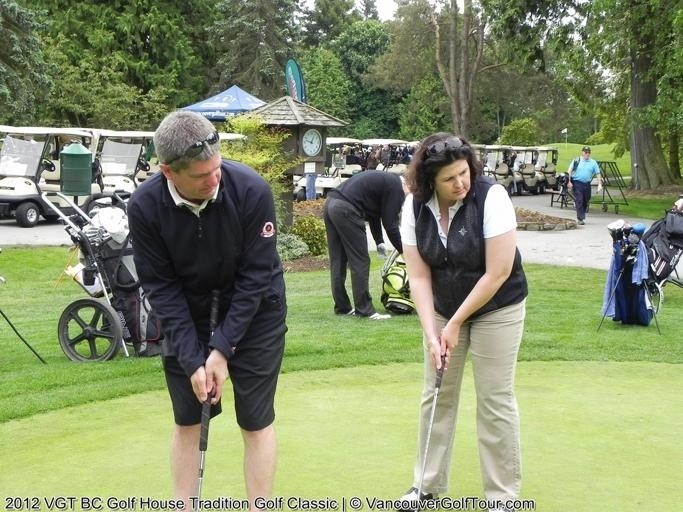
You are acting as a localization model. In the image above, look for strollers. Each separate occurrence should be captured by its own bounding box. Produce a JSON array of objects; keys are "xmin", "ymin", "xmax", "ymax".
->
[{"xmin": 38, "ymin": 191, "xmax": 159, "ymax": 365}]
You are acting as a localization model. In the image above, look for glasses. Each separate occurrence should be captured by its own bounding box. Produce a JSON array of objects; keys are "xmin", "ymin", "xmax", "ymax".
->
[
  {"xmin": 421, "ymin": 136, "xmax": 467, "ymax": 162},
  {"xmin": 584, "ymin": 151, "xmax": 590, "ymax": 154},
  {"xmin": 167, "ymin": 130, "xmax": 219, "ymax": 167}
]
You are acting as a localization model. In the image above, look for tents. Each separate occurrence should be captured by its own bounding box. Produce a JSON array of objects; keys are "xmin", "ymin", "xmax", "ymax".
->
[{"xmin": 180, "ymin": 85, "xmax": 267, "ymax": 120}]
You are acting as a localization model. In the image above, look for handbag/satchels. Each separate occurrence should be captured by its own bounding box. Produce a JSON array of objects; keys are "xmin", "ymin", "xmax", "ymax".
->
[
  {"xmin": 555, "ymin": 172, "xmax": 569, "ymax": 187},
  {"xmin": 381, "ymin": 255, "xmax": 416, "ymax": 314}
]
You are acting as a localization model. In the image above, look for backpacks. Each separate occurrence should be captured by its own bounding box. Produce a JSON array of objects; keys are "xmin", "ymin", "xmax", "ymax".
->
[{"xmin": 376, "ymin": 246, "xmax": 419, "ymax": 315}]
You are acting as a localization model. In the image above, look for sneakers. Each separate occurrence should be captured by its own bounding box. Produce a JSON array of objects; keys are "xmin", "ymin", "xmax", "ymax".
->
[
  {"xmin": 578, "ymin": 220, "xmax": 584, "ymax": 225},
  {"xmin": 399, "ymin": 487, "xmax": 440, "ymax": 512},
  {"xmin": 347, "ymin": 309, "xmax": 356, "ymax": 316},
  {"xmin": 369, "ymin": 313, "xmax": 392, "ymax": 320}
]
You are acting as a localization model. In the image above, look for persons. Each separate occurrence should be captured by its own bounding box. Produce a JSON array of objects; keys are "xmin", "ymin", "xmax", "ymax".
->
[
  {"xmin": 127, "ymin": 112, "xmax": 288, "ymax": 511},
  {"xmin": 567, "ymin": 146, "xmax": 602, "ymax": 225},
  {"xmin": 400, "ymin": 133, "xmax": 529, "ymax": 510},
  {"xmin": 323, "ymin": 170, "xmax": 407, "ymax": 319}
]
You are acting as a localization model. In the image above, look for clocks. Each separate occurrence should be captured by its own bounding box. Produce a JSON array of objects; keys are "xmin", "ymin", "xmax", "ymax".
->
[{"xmin": 302, "ymin": 128, "xmax": 322, "ymax": 156}]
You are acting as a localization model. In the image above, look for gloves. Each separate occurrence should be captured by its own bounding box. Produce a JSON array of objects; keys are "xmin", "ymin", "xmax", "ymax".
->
[{"xmin": 377, "ymin": 243, "xmax": 393, "ymax": 257}]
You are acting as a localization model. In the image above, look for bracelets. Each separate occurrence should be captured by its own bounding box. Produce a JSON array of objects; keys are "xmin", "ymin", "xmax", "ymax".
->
[{"xmin": 427, "ymin": 336, "xmax": 439, "ymax": 348}]
